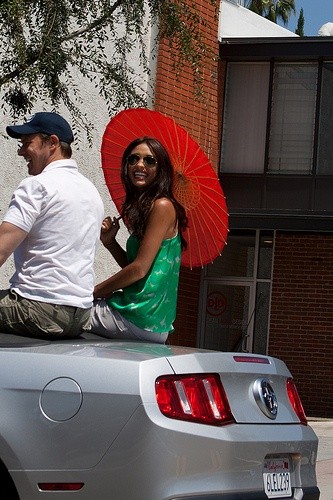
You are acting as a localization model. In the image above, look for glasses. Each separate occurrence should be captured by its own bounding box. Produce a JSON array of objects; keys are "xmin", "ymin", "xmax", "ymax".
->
[{"xmin": 125, "ymin": 154, "xmax": 157, "ymax": 168}]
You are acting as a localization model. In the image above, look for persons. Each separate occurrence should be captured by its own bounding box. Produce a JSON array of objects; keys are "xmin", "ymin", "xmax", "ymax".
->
[
  {"xmin": 88, "ymin": 136, "xmax": 188, "ymax": 346},
  {"xmin": 0, "ymin": 110, "xmax": 104, "ymax": 340}
]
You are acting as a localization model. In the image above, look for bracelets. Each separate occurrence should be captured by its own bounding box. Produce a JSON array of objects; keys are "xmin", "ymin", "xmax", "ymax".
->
[{"xmin": 105, "ymin": 239, "xmax": 116, "ymax": 248}]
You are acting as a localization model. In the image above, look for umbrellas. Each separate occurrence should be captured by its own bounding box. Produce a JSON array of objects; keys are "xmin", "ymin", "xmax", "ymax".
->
[{"xmin": 98, "ymin": 108, "xmax": 231, "ymax": 270}]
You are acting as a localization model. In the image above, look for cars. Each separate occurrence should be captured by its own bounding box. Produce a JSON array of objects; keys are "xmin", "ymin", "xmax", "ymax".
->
[{"xmin": 1, "ymin": 330, "xmax": 321, "ymax": 500}]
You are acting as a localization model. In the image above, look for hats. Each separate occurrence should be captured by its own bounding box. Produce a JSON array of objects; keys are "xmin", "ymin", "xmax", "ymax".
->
[{"xmin": 6, "ymin": 112, "xmax": 74, "ymax": 144}]
